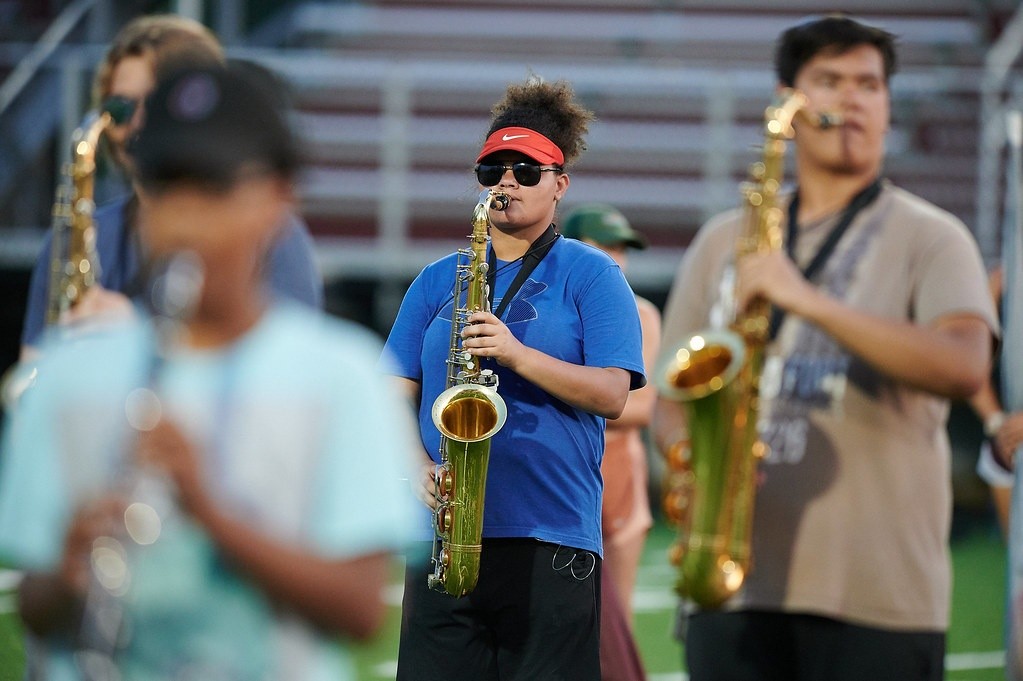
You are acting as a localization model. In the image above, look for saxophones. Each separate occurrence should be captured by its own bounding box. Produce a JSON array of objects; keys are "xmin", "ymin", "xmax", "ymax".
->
[
  {"xmin": 425, "ymin": 185, "xmax": 514, "ymax": 601},
  {"xmin": 44, "ymin": 109, "xmax": 116, "ymax": 331},
  {"xmin": 655, "ymin": 89, "xmax": 815, "ymax": 611}
]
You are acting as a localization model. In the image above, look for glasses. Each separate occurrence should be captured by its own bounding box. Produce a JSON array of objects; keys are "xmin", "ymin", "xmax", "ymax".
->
[
  {"xmin": 474, "ymin": 161, "xmax": 563, "ymax": 187},
  {"xmin": 101, "ymin": 95, "xmax": 152, "ymax": 126}
]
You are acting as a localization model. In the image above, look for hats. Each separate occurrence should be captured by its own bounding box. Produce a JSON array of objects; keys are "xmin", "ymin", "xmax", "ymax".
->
[
  {"xmin": 564, "ymin": 207, "xmax": 647, "ymax": 252},
  {"xmin": 128, "ymin": 58, "xmax": 294, "ymax": 175},
  {"xmin": 476, "ymin": 127, "xmax": 565, "ymax": 167}
]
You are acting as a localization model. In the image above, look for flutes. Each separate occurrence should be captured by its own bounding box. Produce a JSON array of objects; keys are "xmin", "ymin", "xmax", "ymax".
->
[{"xmin": 60, "ymin": 247, "xmax": 203, "ymax": 679}]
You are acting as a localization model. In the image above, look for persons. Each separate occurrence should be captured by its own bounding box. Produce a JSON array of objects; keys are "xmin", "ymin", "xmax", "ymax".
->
[
  {"xmin": 563, "ymin": 203, "xmax": 662, "ymax": 681},
  {"xmin": 379, "ymin": 77, "xmax": 643, "ymax": 681},
  {"xmin": 0, "ymin": 54, "xmax": 431, "ymax": 681},
  {"xmin": 22, "ymin": 11, "xmax": 321, "ymax": 360},
  {"xmin": 971, "ymin": 261, "xmax": 1023, "ymax": 525},
  {"xmin": 653, "ymin": 18, "xmax": 1002, "ymax": 681}
]
